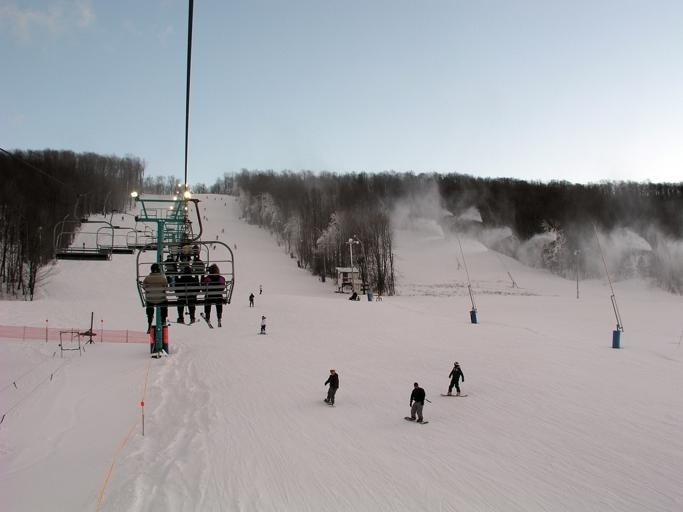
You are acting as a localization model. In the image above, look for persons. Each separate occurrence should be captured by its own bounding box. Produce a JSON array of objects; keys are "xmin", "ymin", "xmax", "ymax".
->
[
  {"xmin": 259, "ymin": 284, "xmax": 263, "ymax": 295},
  {"xmin": 258, "ymin": 315, "xmax": 267, "ymax": 334},
  {"xmin": 322, "ymin": 369, "xmax": 340, "ymax": 406},
  {"xmin": 163, "ymin": 232, "xmax": 209, "ymax": 292},
  {"xmin": 141, "ymin": 262, "xmax": 169, "ymax": 334},
  {"xmin": 248, "ymin": 292, "xmax": 256, "ymax": 307},
  {"xmin": 174, "ymin": 265, "xmax": 200, "ymax": 326},
  {"xmin": 348, "ymin": 289, "xmax": 358, "ymax": 301},
  {"xmin": 201, "ymin": 263, "xmax": 227, "ymax": 323},
  {"xmin": 445, "ymin": 361, "xmax": 465, "ymax": 396},
  {"xmin": 409, "ymin": 382, "xmax": 426, "ymax": 423}
]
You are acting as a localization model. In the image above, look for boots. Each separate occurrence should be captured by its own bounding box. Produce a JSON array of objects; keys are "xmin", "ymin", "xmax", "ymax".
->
[
  {"xmin": 411, "ymin": 417, "xmax": 423, "ymax": 422},
  {"xmin": 326, "ymin": 398, "xmax": 334, "ymax": 404},
  {"xmin": 448, "ymin": 391, "xmax": 460, "ymax": 396}
]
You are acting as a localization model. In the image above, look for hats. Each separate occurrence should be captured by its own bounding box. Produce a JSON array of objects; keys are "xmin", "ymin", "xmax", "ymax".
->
[{"xmin": 455, "ymin": 362, "xmax": 460, "ymax": 365}]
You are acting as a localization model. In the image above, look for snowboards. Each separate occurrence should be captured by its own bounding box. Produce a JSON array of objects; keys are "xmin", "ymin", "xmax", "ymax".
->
[
  {"xmin": 405, "ymin": 417, "xmax": 428, "ymax": 424},
  {"xmin": 440, "ymin": 393, "xmax": 467, "ymax": 397},
  {"xmin": 324, "ymin": 399, "xmax": 333, "ymax": 406}
]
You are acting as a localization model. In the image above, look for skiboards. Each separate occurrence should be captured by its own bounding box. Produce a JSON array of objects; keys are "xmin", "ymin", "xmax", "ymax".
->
[
  {"xmin": 167, "ymin": 316, "xmax": 200, "ymax": 327},
  {"xmin": 147, "ymin": 316, "xmax": 169, "ymax": 334},
  {"xmin": 200, "ymin": 312, "xmax": 222, "ymax": 328}
]
